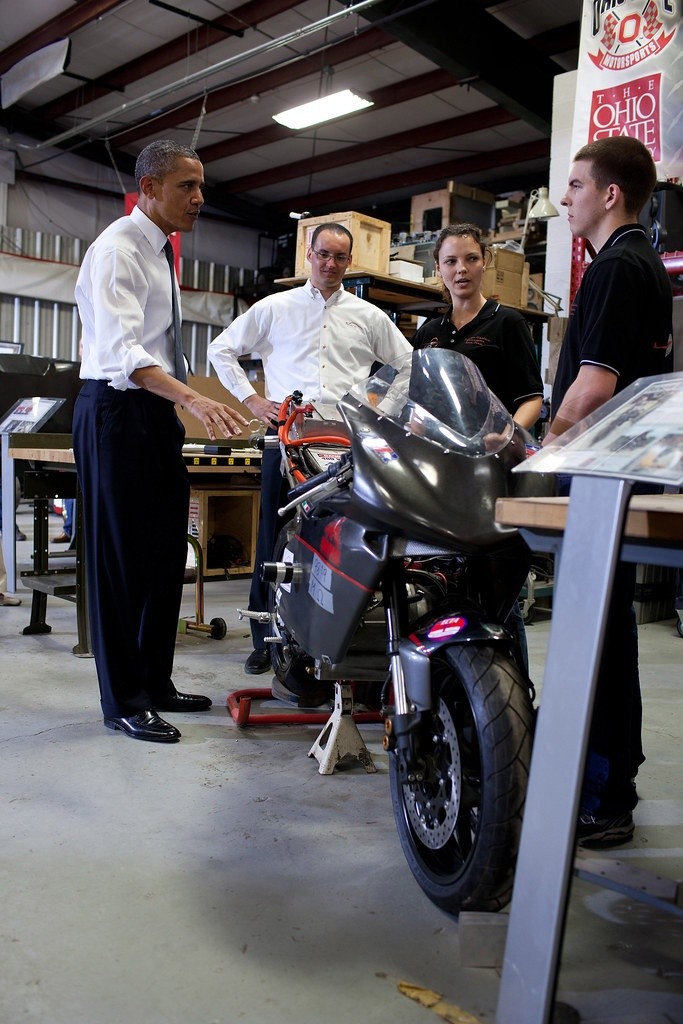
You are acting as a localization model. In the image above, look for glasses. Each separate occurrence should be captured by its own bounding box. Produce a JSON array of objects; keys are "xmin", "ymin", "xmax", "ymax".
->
[{"xmin": 313, "ymin": 251, "xmax": 347, "ymax": 262}]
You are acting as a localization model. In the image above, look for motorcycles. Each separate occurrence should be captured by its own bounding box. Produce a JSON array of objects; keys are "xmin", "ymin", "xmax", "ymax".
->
[{"xmin": 237, "ymin": 348, "xmax": 533, "ymax": 911}]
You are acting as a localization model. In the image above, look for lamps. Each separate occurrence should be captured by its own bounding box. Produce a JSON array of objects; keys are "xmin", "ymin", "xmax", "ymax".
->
[
  {"xmin": 520, "ymin": 187, "xmax": 559, "ymax": 247},
  {"xmin": 270, "ymin": 88, "xmax": 375, "ymax": 130}
]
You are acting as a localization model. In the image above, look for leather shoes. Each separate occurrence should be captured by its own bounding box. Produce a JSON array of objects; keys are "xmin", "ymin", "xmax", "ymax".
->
[
  {"xmin": 103, "ymin": 710, "xmax": 182, "ymax": 742},
  {"xmin": 245, "ymin": 648, "xmax": 271, "ymax": 675},
  {"xmin": 149, "ymin": 690, "xmax": 212, "ymax": 713}
]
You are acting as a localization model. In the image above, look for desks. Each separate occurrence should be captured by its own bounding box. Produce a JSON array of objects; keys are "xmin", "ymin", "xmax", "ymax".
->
[
  {"xmin": 9, "ymin": 448, "xmax": 263, "ymax": 659},
  {"xmin": 496, "ymin": 493, "xmax": 683, "ymax": 595}
]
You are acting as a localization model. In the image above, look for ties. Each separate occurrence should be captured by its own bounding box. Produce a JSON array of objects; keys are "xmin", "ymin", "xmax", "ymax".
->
[{"xmin": 163, "ymin": 240, "xmax": 187, "ymax": 410}]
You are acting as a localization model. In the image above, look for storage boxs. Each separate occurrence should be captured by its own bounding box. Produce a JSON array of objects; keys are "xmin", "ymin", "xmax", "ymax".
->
[
  {"xmin": 480, "ymin": 246, "xmax": 524, "ymax": 307},
  {"xmin": 295, "ymin": 211, "xmax": 392, "ymax": 275},
  {"xmin": 389, "ymin": 260, "xmax": 423, "ymax": 282}
]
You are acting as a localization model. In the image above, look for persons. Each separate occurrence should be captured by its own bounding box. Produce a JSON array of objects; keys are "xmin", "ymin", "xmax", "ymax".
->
[
  {"xmin": 207, "ymin": 223, "xmax": 414, "ymax": 674},
  {"xmin": 578, "ymin": 391, "xmax": 683, "ymax": 473},
  {"xmin": 2, "ymin": 404, "xmax": 36, "ymax": 433},
  {"xmin": 413, "ymin": 224, "xmax": 545, "ymax": 680},
  {"xmin": 540, "ymin": 136, "xmax": 672, "ymax": 847},
  {"xmin": 0, "ymin": 476, "xmax": 27, "ymax": 541},
  {"xmin": 51, "ymin": 499, "xmax": 76, "ymax": 543},
  {"xmin": 74, "ymin": 140, "xmax": 249, "ymax": 742}
]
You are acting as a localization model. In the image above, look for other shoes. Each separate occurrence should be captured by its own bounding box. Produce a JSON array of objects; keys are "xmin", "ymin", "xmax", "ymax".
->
[{"xmin": 575, "ymin": 813, "xmax": 634, "ymax": 850}]
